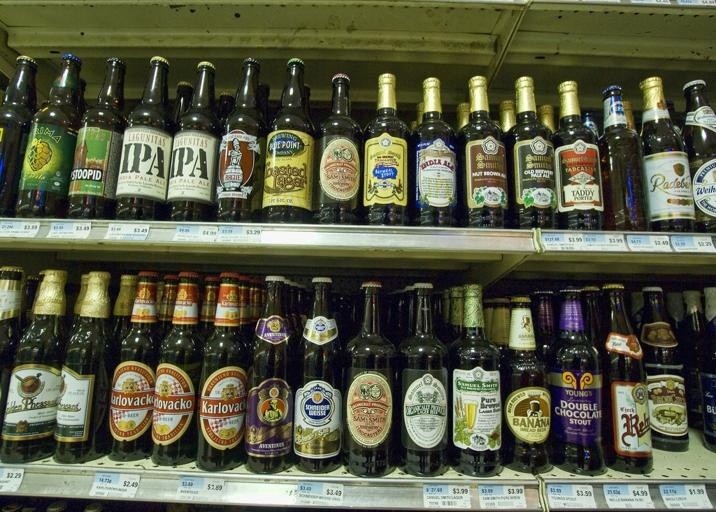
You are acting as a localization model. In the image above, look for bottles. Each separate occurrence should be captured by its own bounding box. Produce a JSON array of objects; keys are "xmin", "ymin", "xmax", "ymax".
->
[
  {"xmin": 622, "ymin": 100, "xmax": 634, "ymax": 131},
  {"xmin": 412, "ymin": 77, "xmax": 458, "ymax": 226},
  {"xmin": 197, "ymin": 273, "xmax": 250, "ymax": 472},
  {"xmin": 502, "ymin": 296, "xmax": 553, "ymax": 475},
  {"xmin": 110, "ymin": 272, "xmax": 161, "ymax": 462},
  {"xmin": 66, "ymin": 57, "xmax": 125, "ymax": 219},
  {"xmin": 152, "ymin": 272, "xmax": 203, "ymax": 466},
  {"xmin": 55, "ymin": 272, "xmax": 113, "ymax": 464},
  {"xmin": 238, "ymin": 275, "xmax": 250, "ymax": 351},
  {"xmin": 71, "ymin": 275, "xmax": 88, "ymax": 337},
  {"xmin": 21, "ymin": 276, "xmax": 40, "ymax": 338},
  {"xmin": 158, "ymin": 276, "xmax": 179, "ymax": 348},
  {"xmin": 505, "ymin": 77, "xmax": 554, "ymax": 228},
  {"xmin": 417, "ymin": 102, "xmax": 425, "ymax": 127},
  {"xmin": 581, "ymin": 287, "xmax": 603, "ymax": 349},
  {"xmin": 640, "ymin": 77, "xmax": 695, "ymax": 232},
  {"xmin": 343, "ymin": 282, "xmax": 398, "ymax": 478},
  {"xmin": 262, "ymin": 59, "xmax": 316, "ymax": 223},
  {"xmin": 0, "ymin": 270, "xmax": 66, "ymax": 464},
  {"xmin": 481, "ymin": 299, "xmax": 494, "ymax": 344},
  {"xmin": 315, "ymin": 74, "xmax": 364, "ymax": 223},
  {"xmin": 217, "ymin": 91, "xmax": 236, "ymax": 132},
  {"xmin": 449, "ymin": 287, "xmax": 464, "ymax": 344},
  {"xmin": 582, "ymin": 112, "xmax": 599, "ymax": 139},
  {"xmin": 292, "ymin": 278, "xmax": 344, "ymax": 474},
  {"xmin": 458, "ymin": 103, "xmax": 470, "ymax": 131},
  {"xmin": 363, "ymin": 74, "xmax": 412, "ymax": 225},
  {"xmin": 0, "ymin": 56, "xmax": 38, "ymax": 217},
  {"xmin": 684, "ymin": 292, "xmax": 707, "ymax": 429},
  {"xmin": 215, "ymin": 58, "xmax": 265, "ymax": 222},
  {"xmin": 553, "ymin": 287, "xmax": 605, "ymax": 475},
  {"xmin": 641, "ymin": 288, "xmax": 690, "ymax": 452},
  {"xmin": 665, "ymin": 288, "xmax": 685, "ymax": 334},
  {"xmin": 538, "ymin": 105, "xmax": 555, "ymax": 133},
  {"xmin": 500, "ymin": 100, "xmax": 517, "ymax": 133},
  {"xmin": 555, "ymin": 81, "xmax": 604, "ymax": 229},
  {"xmin": 244, "ymin": 276, "xmax": 293, "ymax": 475},
  {"xmin": 603, "ymin": 284, "xmax": 653, "ymax": 474},
  {"xmin": 681, "ymin": 80, "xmax": 716, "ymax": 232},
  {"xmin": 532, "ymin": 290, "xmax": 555, "ymax": 354},
  {"xmin": 113, "ymin": 275, "xmax": 137, "ymax": 366},
  {"xmin": 198, "ymin": 276, "xmax": 219, "ymax": 341},
  {"xmin": 397, "ymin": 283, "xmax": 451, "ymax": 476},
  {"xmin": 166, "ymin": 62, "xmax": 221, "ymax": 221},
  {"xmin": 172, "ymin": 82, "xmax": 195, "ymax": 126},
  {"xmin": 449, "ymin": 285, "xmax": 505, "ymax": 477},
  {"xmin": 0, "ymin": 265, "xmax": 22, "ymax": 435},
  {"xmin": 490, "ymin": 299, "xmax": 511, "ymax": 352},
  {"xmin": 115, "ymin": 56, "xmax": 174, "ymax": 220},
  {"xmin": 699, "ymin": 287, "xmax": 716, "ymax": 452},
  {"xmin": 16, "ymin": 54, "xmax": 80, "ymax": 218},
  {"xmin": 458, "ymin": 76, "xmax": 507, "ymax": 228},
  {"xmin": 596, "ymin": 85, "xmax": 649, "ymax": 231}
]
